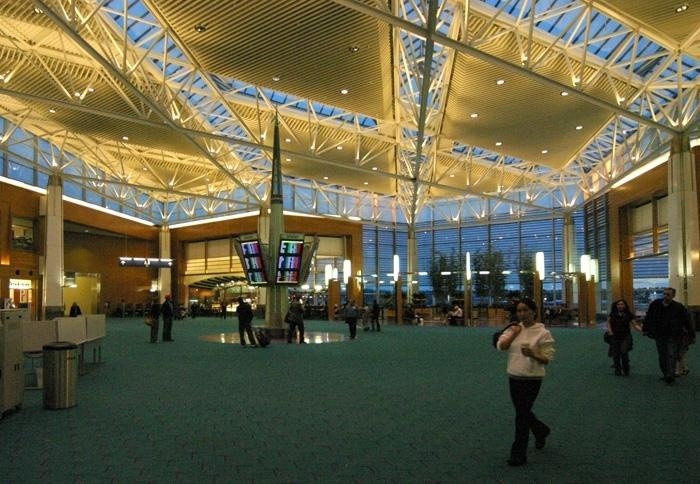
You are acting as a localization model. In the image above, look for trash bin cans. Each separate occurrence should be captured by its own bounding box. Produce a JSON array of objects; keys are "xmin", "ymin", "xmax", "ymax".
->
[{"xmin": 42, "ymin": 341, "xmax": 78, "ymax": 409}]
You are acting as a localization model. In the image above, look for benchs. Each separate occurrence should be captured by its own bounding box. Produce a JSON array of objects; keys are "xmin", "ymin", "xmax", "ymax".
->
[{"xmin": 384, "ymin": 302, "xmax": 511, "ymax": 325}]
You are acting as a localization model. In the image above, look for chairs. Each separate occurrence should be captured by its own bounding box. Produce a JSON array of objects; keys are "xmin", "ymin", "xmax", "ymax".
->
[{"xmin": 21, "ymin": 312, "xmax": 107, "ymax": 390}]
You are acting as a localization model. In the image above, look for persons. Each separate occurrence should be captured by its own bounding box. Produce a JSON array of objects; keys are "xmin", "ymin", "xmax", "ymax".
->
[
  {"xmin": 286, "ymin": 292, "xmax": 307, "ymax": 345},
  {"xmin": 343, "ymin": 298, "xmax": 380, "ymax": 340},
  {"xmin": 69, "ymin": 302, "xmax": 81, "ymax": 317},
  {"xmin": 160, "ymin": 293, "xmax": 175, "ymax": 341},
  {"xmin": 149, "ymin": 299, "xmax": 161, "ymax": 343},
  {"xmin": 497, "ymin": 298, "xmax": 556, "ymax": 467},
  {"xmin": 220, "ymin": 299, "xmax": 227, "ymax": 319},
  {"xmin": 448, "ymin": 305, "xmax": 463, "ymax": 326},
  {"xmin": 190, "ymin": 302, "xmax": 197, "ymax": 318},
  {"xmin": 235, "ymin": 296, "xmax": 256, "ymax": 348},
  {"xmin": 606, "ymin": 287, "xmax": 696, "ymax": 385}
]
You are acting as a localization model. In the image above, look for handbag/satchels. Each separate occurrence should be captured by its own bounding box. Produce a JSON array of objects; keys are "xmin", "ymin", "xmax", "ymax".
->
[
  {"xmin": 493, "ymin": 324, "xmax": 521, "ymax": 349},
  {"xmin": 604, "ymin": 331, "xmax": 614, "ymax": 344},
  {"xmin": 284, "ymin": 311, "xmax": 290, "ymax": 324}
]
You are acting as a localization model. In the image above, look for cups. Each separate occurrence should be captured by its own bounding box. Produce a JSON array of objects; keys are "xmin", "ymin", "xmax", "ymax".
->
[{"xmin": 520, "ymin": 341, "xmax": 529, "ymax": 348}]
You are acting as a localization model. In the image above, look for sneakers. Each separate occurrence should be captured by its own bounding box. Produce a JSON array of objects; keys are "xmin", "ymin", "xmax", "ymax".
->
[
  {"xmin": 507, "ymin": 455, "xmax": 528, "ymax": 467},
  {"xmin": 535, "ymin": 426, "xmax": 551, "ymax": 450}
]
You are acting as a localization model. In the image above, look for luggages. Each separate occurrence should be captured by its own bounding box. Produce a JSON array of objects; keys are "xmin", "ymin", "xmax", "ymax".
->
[{"xmin": 255, "ymin": 328, "xmax": 271, "ymax": 348}]
